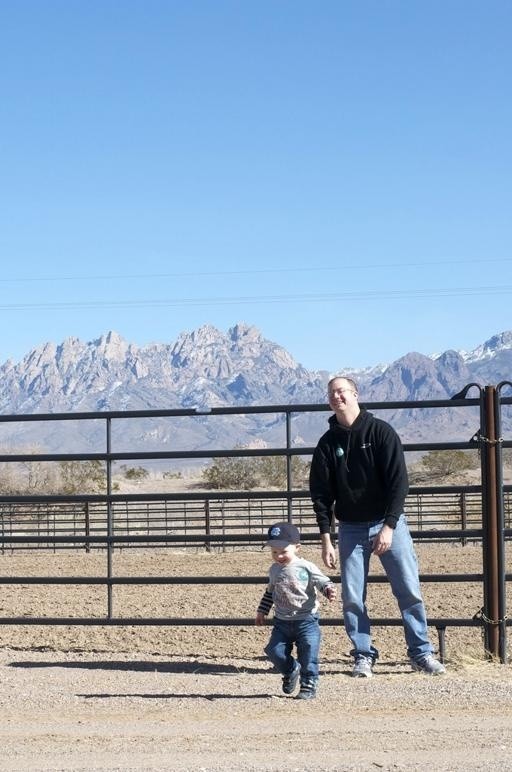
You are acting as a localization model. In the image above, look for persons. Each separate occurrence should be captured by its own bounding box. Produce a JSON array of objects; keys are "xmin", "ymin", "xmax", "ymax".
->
[
  {"xmin": 310, "ymin": 375, "xmax": 446, "ymax": 676},
  {"xmin": 255, "ymin": 521, "xmax": 337, "ymax": 701}
]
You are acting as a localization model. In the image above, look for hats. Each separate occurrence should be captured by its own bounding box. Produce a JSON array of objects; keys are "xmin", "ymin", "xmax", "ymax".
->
[{"xmin": 261, "ymin": 522, "xmax": 300, "ymax": 550}]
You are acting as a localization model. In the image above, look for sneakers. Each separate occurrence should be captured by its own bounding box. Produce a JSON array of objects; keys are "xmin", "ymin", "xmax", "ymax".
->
[
  {"xmin": 352, "ymin": 655, "xmax": 373, "ymax": 678},
  {"xmin": 295, "ymin": 686, "xmax": 316, "ymax": 698},
  {"xmin": 412, "ymin": 654, "xmax": 446, "ymax": 674},
  {"xmin": 282, "ymin": 661, "xmax": 301, "ymax": 692}
]
